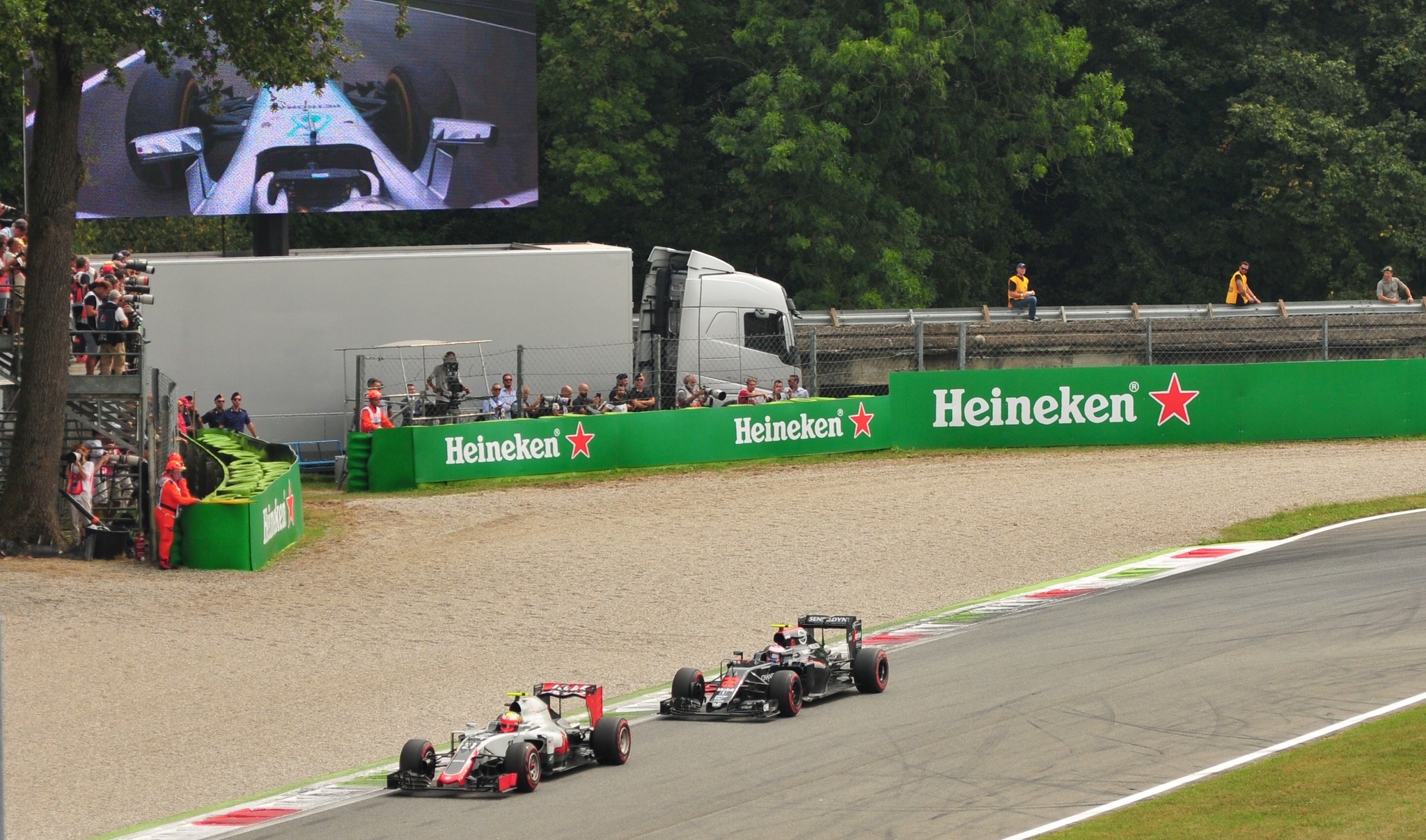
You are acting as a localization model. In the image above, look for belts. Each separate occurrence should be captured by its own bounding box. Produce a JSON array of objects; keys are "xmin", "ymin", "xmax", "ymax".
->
[{"xmin": 159, "ymin": 503, "xmax": 176, "ymax": 512}]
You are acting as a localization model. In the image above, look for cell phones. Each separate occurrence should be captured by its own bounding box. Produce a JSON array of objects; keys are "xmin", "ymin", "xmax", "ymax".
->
[{"xmin": 596, "ymin": 392, "xmax": 601, "ymax": 399}]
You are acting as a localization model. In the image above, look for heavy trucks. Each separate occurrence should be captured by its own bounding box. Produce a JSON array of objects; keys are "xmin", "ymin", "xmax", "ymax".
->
[{"xmin": 136, "ymin": 239, "xmax": 809, "ymax": 463}]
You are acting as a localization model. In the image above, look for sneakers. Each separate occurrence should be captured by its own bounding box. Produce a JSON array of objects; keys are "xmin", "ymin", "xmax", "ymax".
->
[{"xmin": 1027, "ymin": 316, "xmax": 1041, "ymax": 322}]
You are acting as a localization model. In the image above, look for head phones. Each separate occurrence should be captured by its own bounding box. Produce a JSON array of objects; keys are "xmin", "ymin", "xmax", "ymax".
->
[{"xmin": 442, "ymin": 352, "xmax": 459, "ymax": 361}]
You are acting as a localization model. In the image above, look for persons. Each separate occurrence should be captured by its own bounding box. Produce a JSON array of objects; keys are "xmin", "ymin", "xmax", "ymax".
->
[
  {"xmin": 0, "ymin": 202, "xmax": 28, "ymax": 335},
  {"xmin": 783, "ymin": 375, "xmax": 809, "ymax": 400},
  {"xmin": 426, "ymin": 351, "xmax": 471, "ymax": 425},
  {"xmin": 69, "ymin": 250, "xmax": 139, "ymax": 375},
  {"xmin": 482, "ymin": 374, "xmax": 541, "ymax": 421},
  {"xmin": 1226, "ymin": 261, "xmax": 1261, "ymax": 307},
  {"xmin": 176, "ymin": 392, "xmax": 259, "ymax": 444},
  {"xmin": 736, "ymin": 378, "xmax": 769, "ymax": 404},
  {"xmin": 552, "ymin": 373, "xmax": 655, "ymax": 416},
  {"xmin": 359, "ymin": 378, "xmax": 396, "ymax": 433},
  {"xmin": 676, "ymin": 374, "xmax": 709, "ymax": 409},
  {"xmin": 154, "ymin": 452, "xmax": 200, "ymax": 572},
  {"xmin": 1377, "ymin": 266, "xmax": 1414, "ymax": 304},
  {"xmin": 1008, "ymin": 263, "xmax": 1042, "ymax": 323},
  {"xmin": 400, "ymin": 383, "xmax": 423, "ymax": 428},
  {"xmin": 65, "ymin": 392, "xmax": 153, "ymax": 546},
  {"xmin": 766, "ymin": 380, "xmax": 787, "ymax": 402},
  {"xmin": 500, "ymin": 711, "xmax": 520, "ymax": 733},
  {"xmin": 766, "ymin": 645, "xmax": 785, "ymax": 664}
]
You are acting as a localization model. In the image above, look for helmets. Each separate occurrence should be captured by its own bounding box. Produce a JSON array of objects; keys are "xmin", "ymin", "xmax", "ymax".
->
[
  {"xmin": 368, "ymin": 390, "xmax": 381, "ymax": 399},
  {"xmin": 165, "ymin": 453, "xmax": 186, "ymax": 471},
  {"xmin": 500, "ymin": 712, "xmax": 520, "ymax": 731},
  {"xmin": 766, "ymin": 645, "xmax": 786, "ymax": 663}
]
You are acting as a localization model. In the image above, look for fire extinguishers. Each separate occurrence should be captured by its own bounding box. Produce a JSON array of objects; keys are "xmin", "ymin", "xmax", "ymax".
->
[{"xmin": 134, "ymin": 535, "xmax": 146, "ymax": 560}]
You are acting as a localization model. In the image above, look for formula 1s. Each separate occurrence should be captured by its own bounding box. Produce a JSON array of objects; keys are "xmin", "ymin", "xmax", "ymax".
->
[
  {"xmin": 385, "ymin": 680, "xmax": 631, "ymax": 795},
  {"xmin": 658, "ymin": 614, "xmax": 890, "ymax": 719}
]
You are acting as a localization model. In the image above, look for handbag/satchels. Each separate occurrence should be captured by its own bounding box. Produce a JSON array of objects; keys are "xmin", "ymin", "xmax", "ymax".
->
[{"xmin": 67, "ymin": 472, "xmax": 83, "ymax": 494}]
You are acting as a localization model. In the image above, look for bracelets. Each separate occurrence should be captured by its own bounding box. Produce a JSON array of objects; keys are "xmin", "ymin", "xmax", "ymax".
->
[
  {"xmin": 196, "ymin": 414, "xmax": 199, "ymax": 417},
  {"xmin": 1021, "ymin": 293, "xmax": 1023, "ymax": 297},
  {"xmin": 1253, "ymin": 296, "xmax": 1256, "ymax": 299},
  {"xmin": 256, "ymin": 436, "xmax": 259, "ymax": 438},
  {"xmin": 1408, "ymin": 295, "xmax": 1412, "ymax": 297}
]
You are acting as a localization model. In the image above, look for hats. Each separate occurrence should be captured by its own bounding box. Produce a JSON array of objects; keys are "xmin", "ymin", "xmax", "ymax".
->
[
  {"xmin": 1017, "ymin": 263, "xmax": 1027, "ymax": 268},
  {"xmin": 214, "ymin": 394, "xmax": 222, "ymax": 401},
  {"xmin": 179, "ymin": 397, "xmax": 191, "ymax": 407},
  {"xmin": 616, "ymin": 374, "xmax": 628, "ymax": 379},
  {"xmin": 186, "ymin": 396, "xmax": 192, "ymax": 401},
  {"xmin": 1381, "ymin": 266, "xmax": 1393, "ymax": 273},
  {"xmin": 231, "ymin": 392, "xmax": 239, "ymax": 400},
  {"xmin": 108, "ymin": 289, "xmax": 122, "ymax": 298},
  {"xmin": 103, "ymin": 250, "xmax": 130, "ymax": 284},
  {"xmin": 634, "ymin": 373, "xmax": 643, "ymax": 379}
]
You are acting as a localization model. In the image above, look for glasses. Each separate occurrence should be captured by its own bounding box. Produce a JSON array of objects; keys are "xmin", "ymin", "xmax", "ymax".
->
[
  {"xmin": 493, "ymin": 388, "xmax": 501, "ymax": 391},
  {"xmin": 233, "ymin": 398, "xmax": 241, "ymax": 401},
  {"xmin": 505, "ymin": 379, "xmax": 512, "ymax": 381},
  {"xmin": 1241, "ymin": 267, "xmax": 1248, "ymax": 271},
  {"xmin": 216, "ymin": 400, "xmax": 224, "ymax": 402},
  {"xmin": 637, "ymin": 379, "xmax": 643, "ymax": 381}
]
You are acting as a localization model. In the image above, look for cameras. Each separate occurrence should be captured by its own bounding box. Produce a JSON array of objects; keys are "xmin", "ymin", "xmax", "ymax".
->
[
  {"xmin": 66, "ymin": 451, "xmax": 83, "ymax": 464},
  {"xmin": 4, "ymin": 205, "xmax": 18, "ymax": 215},
  {"xmin": 124, "ymin": 284, "xmax": 151, "ymax": 294},
  {"xmin": 106, "ymin": 453, "xmax": 140, "ymax": 465},
  {"xmin": 123, "ymin": 293, "xmax": 154, "ymax": 305},
  {"xmin": 700, "ymin": 385, "xmax": 727, "ymax": 401},
  {"xmin": 122, "ymin": 258, "xmax": 156, "ymax": 274},
  {"xmin": 541, "ymin": 395, "xmax": 571, "ymax": 406},
  {"xmin": 124, "ymin": 275, "xmax": 149, "ymax": 286}
]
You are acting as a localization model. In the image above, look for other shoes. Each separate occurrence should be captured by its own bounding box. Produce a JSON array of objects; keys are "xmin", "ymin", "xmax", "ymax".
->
[
  {"xmin": 161, "ymin": 562, "xmax": 176, "ymax": 570},
  {"xmin": 76, "ymin": 354, "xmax": 89, "ymax": 363},
  {"xmin": 71, "ymin": 541, "xmax": 78, "ymax": 546},
  {"xmin": 80, "ymin": 538, "xmax": 86, "ymax": 545}
]
